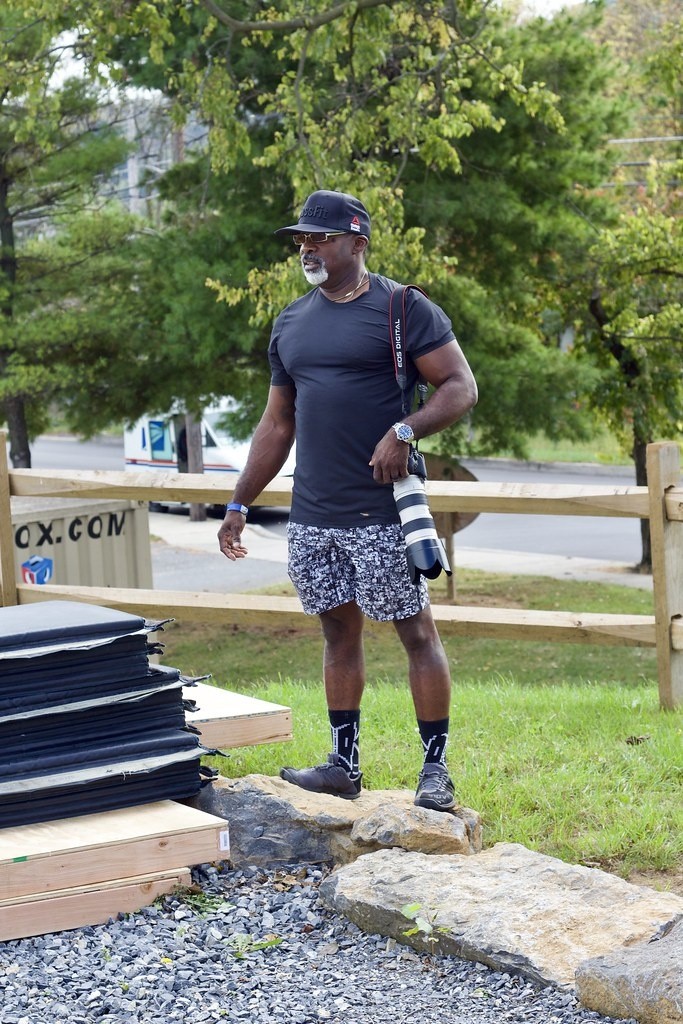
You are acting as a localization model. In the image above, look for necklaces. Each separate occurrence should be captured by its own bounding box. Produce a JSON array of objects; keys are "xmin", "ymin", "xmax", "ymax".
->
[{"xmin": 332, "ymin": 270, "xmax": 370, "ymax": 303}]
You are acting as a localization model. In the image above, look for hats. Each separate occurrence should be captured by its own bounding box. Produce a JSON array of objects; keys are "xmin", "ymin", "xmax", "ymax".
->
[{"xmin": 274, "ymin": 189, "xmax": 371, "ymax": 240}]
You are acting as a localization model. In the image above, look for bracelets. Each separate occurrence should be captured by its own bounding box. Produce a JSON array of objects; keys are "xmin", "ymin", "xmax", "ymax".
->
[{"xmin": 226, "ymin": 503, "xmax": 248, "ymax": 516}]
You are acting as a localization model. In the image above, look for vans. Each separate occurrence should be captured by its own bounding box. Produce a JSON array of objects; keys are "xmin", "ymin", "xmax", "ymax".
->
[{"xmin": 124, "ymin": 391, "xmax": 297, "ymax": 517}]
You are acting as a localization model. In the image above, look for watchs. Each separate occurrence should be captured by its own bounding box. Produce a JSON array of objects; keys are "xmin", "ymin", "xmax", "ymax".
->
[{"xmin": 390, "ymin": 422, "xmax": 415, "ymax": 445}]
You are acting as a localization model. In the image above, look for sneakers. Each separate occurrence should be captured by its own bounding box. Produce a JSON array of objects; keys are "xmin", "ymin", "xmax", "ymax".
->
[
  {"xmin": 414, "ymin": 763, "xmax": 455, "ymax": 811},
  {"xmin": 280, "ymin": 753, "xmax": 363, "ymax": 799}
]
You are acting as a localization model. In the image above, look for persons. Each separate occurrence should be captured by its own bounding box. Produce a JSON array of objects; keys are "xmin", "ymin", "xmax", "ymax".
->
[{"xmin": 218, "ymin": 191, "xmax": 480, "ymax": 811}]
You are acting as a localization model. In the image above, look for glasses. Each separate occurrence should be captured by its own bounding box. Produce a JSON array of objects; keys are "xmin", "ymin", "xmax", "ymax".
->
[{"xmin": 293, "ymin": 232, "xmax": 349, "ymax": 245}]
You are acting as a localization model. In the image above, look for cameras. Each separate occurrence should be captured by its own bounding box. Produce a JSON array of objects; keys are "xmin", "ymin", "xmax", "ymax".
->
[{"xmin": 391, "ymin": 449, "xmax": 453, "ymax": 585}]
